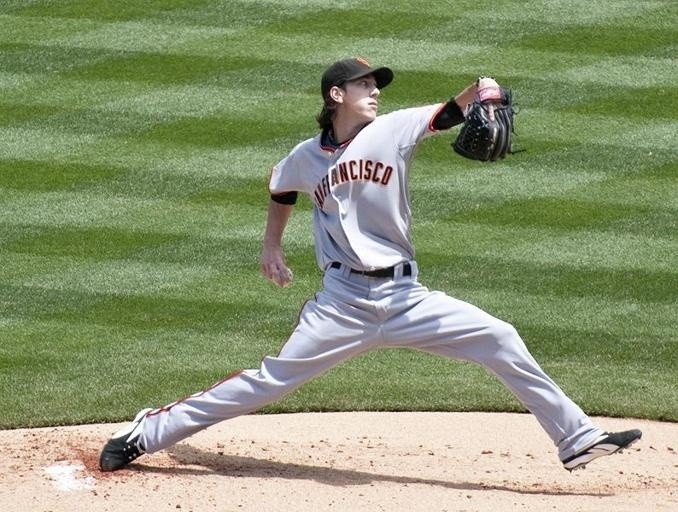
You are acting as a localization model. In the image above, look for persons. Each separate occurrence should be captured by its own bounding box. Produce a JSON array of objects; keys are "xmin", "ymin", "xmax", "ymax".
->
[{"xmin": 95, "ymin": 56, "xmax": 643, "ymax": 478}]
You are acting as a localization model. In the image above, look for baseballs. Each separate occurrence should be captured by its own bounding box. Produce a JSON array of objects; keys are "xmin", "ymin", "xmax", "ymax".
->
[{"xmin": 273, "ymin": 268, "xmax": 292, "ymax": 286}]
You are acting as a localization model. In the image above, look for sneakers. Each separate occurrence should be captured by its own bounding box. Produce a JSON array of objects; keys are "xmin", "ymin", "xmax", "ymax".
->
[
  {"xmin": 562, "ymin": 429, "xmax": 642, "ymax": 472},
  {"xmin": 99, "ymin": 407, "xmax": 155, "ymax": 472}
]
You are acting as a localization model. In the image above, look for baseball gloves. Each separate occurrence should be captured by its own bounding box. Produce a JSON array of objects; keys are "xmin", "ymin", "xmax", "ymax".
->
[{"xmin": 451, "ymin": 76, "xmax": 527, "ymax": 162}]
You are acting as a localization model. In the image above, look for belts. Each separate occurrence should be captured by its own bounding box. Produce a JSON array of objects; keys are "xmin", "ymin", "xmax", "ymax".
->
[{"xmin": 330, "ymin": 261, "xmax": 421, "ymax": 277}]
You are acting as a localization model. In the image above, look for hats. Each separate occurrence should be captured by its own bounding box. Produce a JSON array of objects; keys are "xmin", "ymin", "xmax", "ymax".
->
[{"xmin": 321, "ymin": 56, "xmax": 394, "ymax": 101}]
